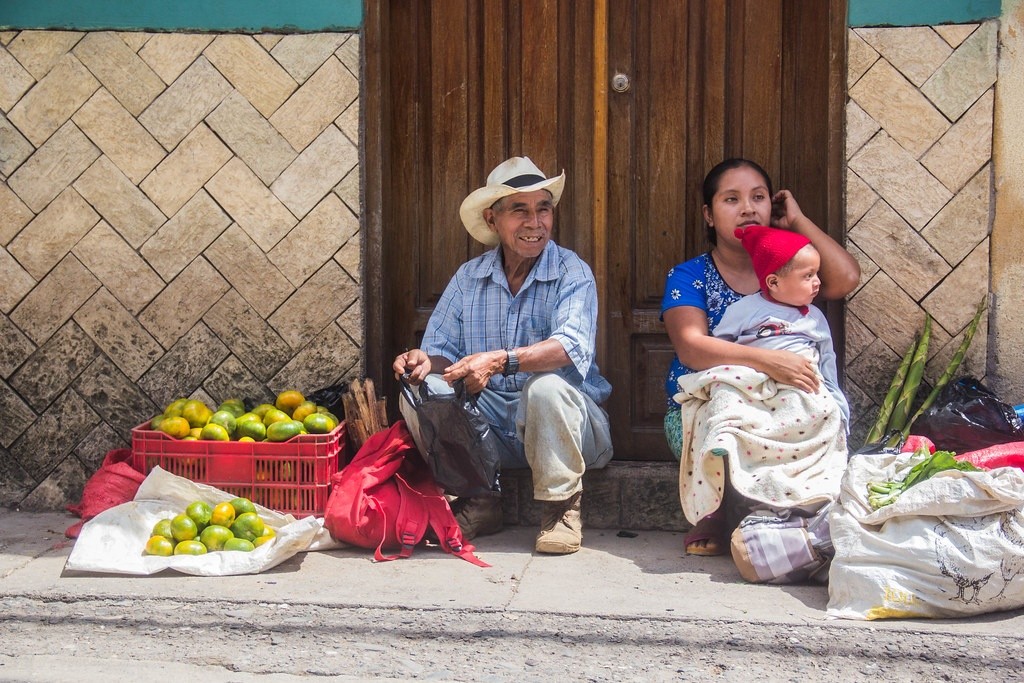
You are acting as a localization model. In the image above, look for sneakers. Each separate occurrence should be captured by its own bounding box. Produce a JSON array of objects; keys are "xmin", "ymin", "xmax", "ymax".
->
[
  {"xmin": 535, "ymin": 489, "xmax": 582, "ymax": 553},
  {"xmin": 429, "ymin": 498, "xmax": 504, "ymax": 541}
]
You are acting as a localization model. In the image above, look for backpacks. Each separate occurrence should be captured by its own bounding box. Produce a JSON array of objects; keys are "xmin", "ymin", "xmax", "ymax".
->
[{"xmin": 324, "ymin": 420, "xmax": 476, "ymax": 567}]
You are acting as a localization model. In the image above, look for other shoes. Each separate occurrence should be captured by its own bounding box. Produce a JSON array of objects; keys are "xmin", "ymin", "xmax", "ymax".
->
[{"xmin": 684, "ymin": 511, "xmax": 729, "ymax": 555}]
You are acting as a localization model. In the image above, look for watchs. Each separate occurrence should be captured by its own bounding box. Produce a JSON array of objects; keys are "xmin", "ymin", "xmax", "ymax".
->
[{"xmin": 502, "ymin": 346, "xmax": 520, "ymax": 378}]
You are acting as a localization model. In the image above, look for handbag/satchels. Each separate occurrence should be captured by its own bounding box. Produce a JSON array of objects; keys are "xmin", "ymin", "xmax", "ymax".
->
[{"xmin": 731, "ymin": 510, "xmax": 831, "ymax": 583}]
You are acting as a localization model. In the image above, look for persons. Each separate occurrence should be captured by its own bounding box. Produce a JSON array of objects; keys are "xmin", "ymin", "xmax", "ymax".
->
[
  {"xmin": 658, "ymin": 157, "xmax": 860, "ymax": 556},
  {"xmin": 711, "ymin": 228, "xmax": 851, "ymax": 456},
  {"xmin": 392, "ymin": 157, "xmax": 616, "ymax": 556}
]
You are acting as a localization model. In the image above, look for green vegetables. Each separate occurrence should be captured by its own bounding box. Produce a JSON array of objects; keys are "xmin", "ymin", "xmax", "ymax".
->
[{"xmin": 867, "ymin": 451, "xmax": 984, "ymax": 511}]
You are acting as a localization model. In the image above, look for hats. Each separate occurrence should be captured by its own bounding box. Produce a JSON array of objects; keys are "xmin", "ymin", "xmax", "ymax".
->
[
  {"xmin": 734, "ymin": 226, "xmax": 812, "ymax": 316},
  {"xmin": 459, "ymin": 156, "xmax": 566, "ymax": 246}
]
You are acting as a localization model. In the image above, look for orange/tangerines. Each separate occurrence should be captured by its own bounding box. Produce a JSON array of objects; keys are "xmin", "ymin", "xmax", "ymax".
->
[
  {"xmin": 146, "ymin": 496, "xmax": 275, "ymax": 557},
  {"xmin": 151, "ymin": 390, "xmax": 339, "ymax": 509}
]
